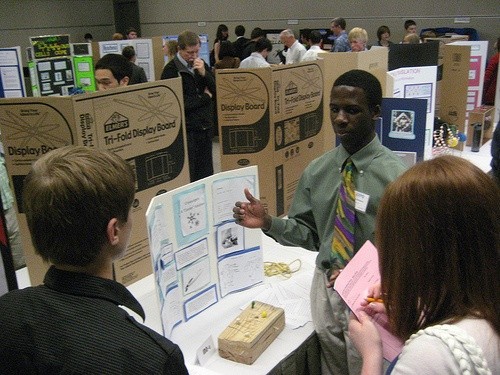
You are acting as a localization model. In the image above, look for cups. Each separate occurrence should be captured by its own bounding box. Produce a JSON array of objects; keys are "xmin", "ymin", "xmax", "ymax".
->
[{"xmin": 471, "ymin": 122, "xmax": 482, "ymax": 152}]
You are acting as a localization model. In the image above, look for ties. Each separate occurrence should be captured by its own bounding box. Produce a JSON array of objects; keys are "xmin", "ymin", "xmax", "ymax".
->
[
  {"xmin": 330, "ymin": 161, "xmax": 356, "ymax": 270},
  {"xmin": 188, "ymin": 63, "xmax": 199, "ymax": 79}
]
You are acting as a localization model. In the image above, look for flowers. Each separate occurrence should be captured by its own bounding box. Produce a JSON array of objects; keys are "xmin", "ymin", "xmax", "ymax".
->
[{"xmin": 432, "ymin": 123, "xmax": 466, "ymax": 158}]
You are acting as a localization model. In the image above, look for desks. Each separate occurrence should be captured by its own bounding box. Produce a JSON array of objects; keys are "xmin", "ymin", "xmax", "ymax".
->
[{"xmin": 16, "ymin": 139, "xmax": 492, "ymax": 375}]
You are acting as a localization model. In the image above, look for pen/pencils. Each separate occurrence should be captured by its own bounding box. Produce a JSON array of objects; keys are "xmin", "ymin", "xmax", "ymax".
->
[{"xmin": 363, "ymin": 297, "xmax": 384, "ymax": 303}]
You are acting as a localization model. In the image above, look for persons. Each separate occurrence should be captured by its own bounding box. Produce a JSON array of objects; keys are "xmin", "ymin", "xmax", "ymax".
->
[
  {"xmin": 279, "ymin": 17, "xmax": 436, "ymax": 65},
  {"xmin": 232, "ymin": 69, "xmax": 411, "ymax": 375},
  {"xmin": 0, "ymin": 146, "xmax": 189, "ymax": 375},
  {"xmin": 84, "ymin": 32, "xmax": 101, "ymax": 68},
  {"xmin": 122, "ymin": 46, "xmax": 148, "ymax": 86},
  {"xmin": 160, "ymin": 32, "xmax": 217, "ymax": 183},
  {"xmin": 347, "ymin": 155, "xmax": 500, "ymax": 375},
  {"xmin": 486, "ymin": 119, "xmax": 500, "ymax": 181},
  {"xmin": 94, "ymin": 53, "xmax": 132, "ymax": 91},
  {"xmin": 112, "ymin": 27, "xmax": 138, "ymax": 43},
  {"xmin": 207, "ymin": 24, "xmax": 273, "ymax": 79},
  {"xmin": 481, "ymin": 37, "xmax": 500, "ymax": 106},
  {"xmin": 163, "ymin": 38, "xmax": 178, "ymax": 63}
]
